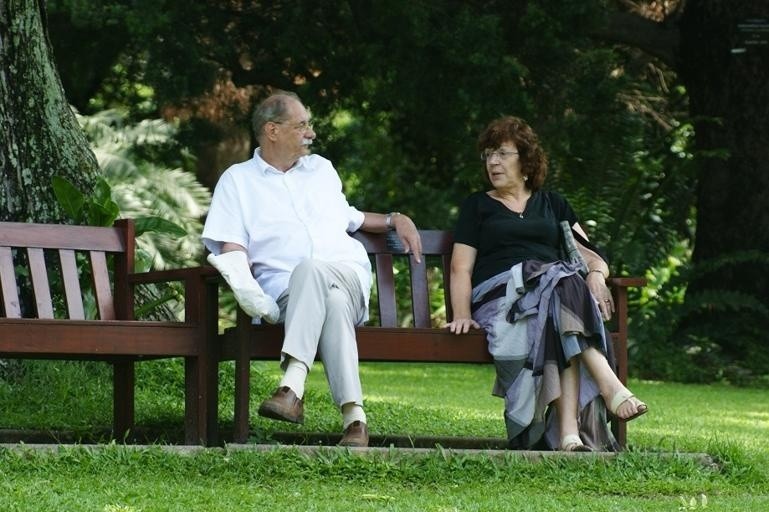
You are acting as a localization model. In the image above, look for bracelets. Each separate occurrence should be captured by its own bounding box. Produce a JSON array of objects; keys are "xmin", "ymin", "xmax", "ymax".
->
[
  {"xmin": 385, "ymin": 211, "xmax": 401, "ymax": 228},
  {"xmin": 590, "ymin": 269, "xmax": 606, "ymax": 280}
]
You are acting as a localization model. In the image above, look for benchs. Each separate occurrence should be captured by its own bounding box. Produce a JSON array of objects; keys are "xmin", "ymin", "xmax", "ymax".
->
[
  {"xmin": 223, "ymin": 228, "xmax": 647, "ymax": 452},
  {"xmin": 0, "ymin": 221, "xmax": 218, "ymax": 448}
]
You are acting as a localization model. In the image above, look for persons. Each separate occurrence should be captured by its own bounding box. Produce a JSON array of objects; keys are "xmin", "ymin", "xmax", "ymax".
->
[
  {"xmin": 199, "ymin": 90, "xmax": 422, "ymax": 447},
  {"xmin": 439, "ymin": 114, "xmax": 648, "ymax": 451}
]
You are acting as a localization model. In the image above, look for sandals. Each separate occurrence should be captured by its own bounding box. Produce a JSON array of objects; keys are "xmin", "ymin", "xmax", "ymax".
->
[
  {"xmin": 559, "ymin": 434, "xmax": 592, "ymax": 452},
  {"xmin": 608, "ymin": 390, "xmax": 648, "ymax": 423}
]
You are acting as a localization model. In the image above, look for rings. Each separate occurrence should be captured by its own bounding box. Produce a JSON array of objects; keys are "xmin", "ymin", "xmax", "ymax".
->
[{"xmin": 604, "ymin": 299, "xmax": 610, "ymax": 303}]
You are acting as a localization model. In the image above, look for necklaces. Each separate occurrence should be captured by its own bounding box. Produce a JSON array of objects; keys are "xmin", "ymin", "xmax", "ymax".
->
[{"xmin": 519, "ymin": 211, "xmax": 526, "ymax": 219}]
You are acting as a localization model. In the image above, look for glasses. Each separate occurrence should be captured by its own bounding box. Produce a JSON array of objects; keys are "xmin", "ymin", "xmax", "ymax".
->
[
  {"xmin": 274, "ymin": 121, "xmax": 314, "ymax": 131},
  {"xmin": 480, "ymin": 149, "xmax": 519, "ymax": 161}
]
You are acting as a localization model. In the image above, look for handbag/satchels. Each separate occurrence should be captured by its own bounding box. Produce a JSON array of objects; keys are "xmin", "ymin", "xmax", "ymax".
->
[{"xmin": 561, "ymin": 220, "xmax": 590, "ymax": 277}]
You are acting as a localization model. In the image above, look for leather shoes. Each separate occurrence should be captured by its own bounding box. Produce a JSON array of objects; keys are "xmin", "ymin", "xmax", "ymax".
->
[
  {"xmin": 338, "ymin": 420, "xmax": 369, "ymax": 447},
  {"xmin": 258, "ymin": 388, "xmax": 304, "ymax": 425}
]
